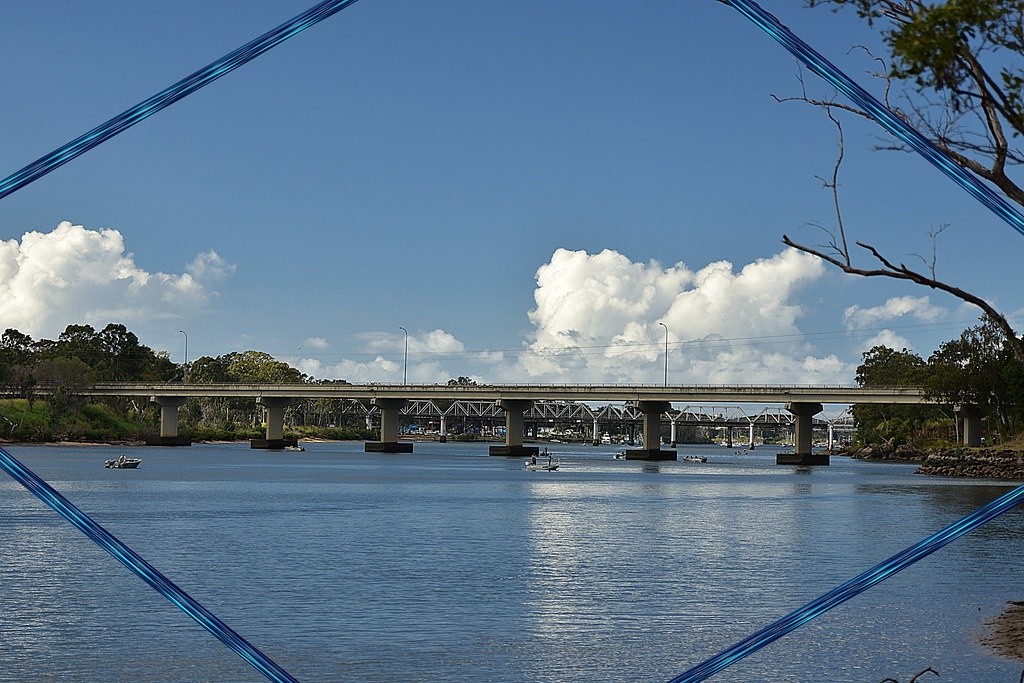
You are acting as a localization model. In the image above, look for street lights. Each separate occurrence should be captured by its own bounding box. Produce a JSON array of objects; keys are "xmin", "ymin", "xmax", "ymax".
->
[
  {"xmin": 399, "ymin": 326, "xmax": 408, "ymax": 386},
  {"xmin": 179, "ymin": 330, "xmax": 187, "ymax": 388},
  {"xmin": 659, "ymin": 323, "xmax": 669, "ymax": 388}
]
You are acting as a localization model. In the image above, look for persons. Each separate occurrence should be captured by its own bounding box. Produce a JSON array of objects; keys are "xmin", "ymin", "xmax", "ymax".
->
[
  {"xmin": 548, "ymin": 453, "xmax": 553, "ymax": 465},
  {"xmin": 119, "ymin": 454, "xmax": 126, "ymax": 465},
  {"xmin": 531, "ymin": 453, "xmax": 537, "ymax": 465},
  {"xmin": 979, "ymin": 435, "xmax": 998, "ymax": 447}
]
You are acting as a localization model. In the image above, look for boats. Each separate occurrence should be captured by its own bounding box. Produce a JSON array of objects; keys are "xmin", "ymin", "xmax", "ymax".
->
[
  {"xmin": 683, "ymin": 455, "xmax": 707, "ymax": 463},
  {"xmin": 524, "ymin": 452, "xmax": 560, "ymax": 473},
  {"xmin": 104, "ymin": 456, "xmax": 141, "ymax": 469},
  {"xmin": 286, "ymin": 447, "xmax": 307, "ymax": 452}
]
